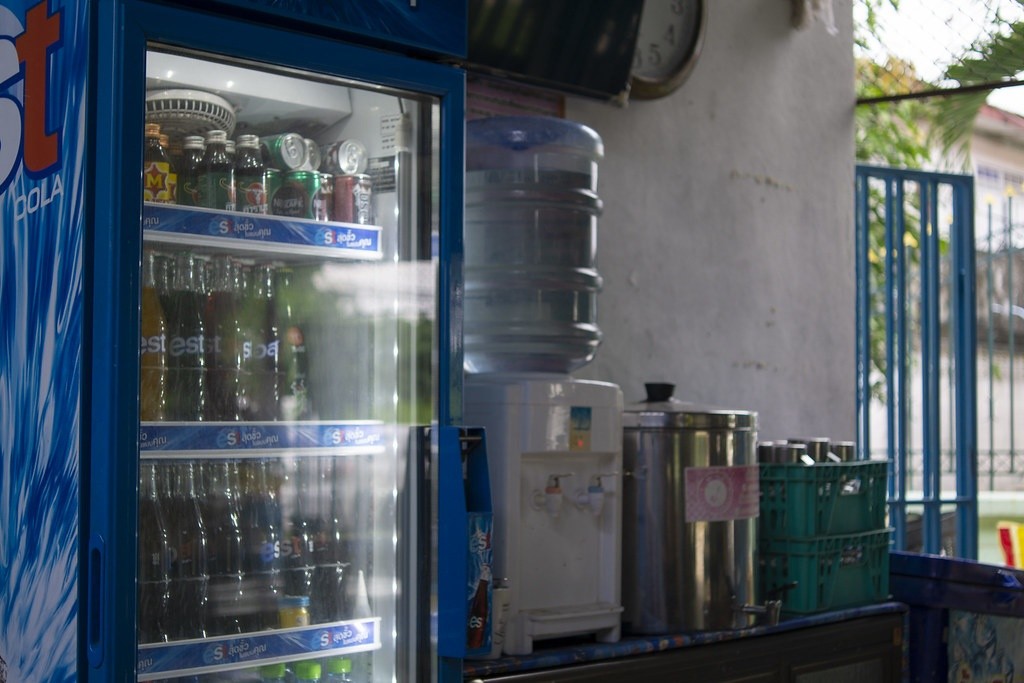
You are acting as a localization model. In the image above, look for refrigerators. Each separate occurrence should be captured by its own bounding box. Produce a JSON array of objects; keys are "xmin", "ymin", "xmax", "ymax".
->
[{"xmin": 0, "ymin": 0, "xmax": 466, "ymax": 683}]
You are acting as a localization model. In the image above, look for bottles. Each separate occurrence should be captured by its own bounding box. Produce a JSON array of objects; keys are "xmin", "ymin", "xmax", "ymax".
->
[
  {"xmin": 258, "ymin": 656, "xmax": 354, "ymax": 683},
  {"xmin": 138, "ymin": 457, "xmax": 350, "ymax": 646},
  {"xmin": 466, "ymin": 564, "xmax": 491, "ymax": 649},
  {"xmin": 144, "ymin": 123, "xmax": 266, "ymax": 214},
  {"xmin": 140, "ymin": 246, "xmax": 313, "ymax": 421},
  {"xmin": 464, "ymin": 116, "xmax": 603, "ymax": 374}
]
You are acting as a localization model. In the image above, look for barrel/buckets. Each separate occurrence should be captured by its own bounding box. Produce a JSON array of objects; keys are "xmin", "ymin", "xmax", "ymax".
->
[{"xmin": 622, "ymin": 382, "xmax": 759, "ymax": 638}]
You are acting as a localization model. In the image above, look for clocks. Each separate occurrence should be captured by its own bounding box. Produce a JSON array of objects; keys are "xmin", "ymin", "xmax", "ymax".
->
[{"xmin": 631, "ymin": 0, "xmax": 709, "ymax": 101}]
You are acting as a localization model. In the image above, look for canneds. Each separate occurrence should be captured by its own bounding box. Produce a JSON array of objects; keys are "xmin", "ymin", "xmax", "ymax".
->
[{"xmin": 259, "ymin": 134, "xmax": 372, "ymax": 228}]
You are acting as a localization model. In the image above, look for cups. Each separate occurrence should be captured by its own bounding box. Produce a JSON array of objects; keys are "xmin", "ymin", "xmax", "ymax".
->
[{"xmin": 759, "ymin": 438, "xmax": 856, "ymax": 464}]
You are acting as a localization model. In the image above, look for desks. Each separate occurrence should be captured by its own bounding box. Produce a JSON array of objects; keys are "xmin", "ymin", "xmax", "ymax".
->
[{"xmin": 458, "ymin": 598, "xmax": 913, "ymax": 683}]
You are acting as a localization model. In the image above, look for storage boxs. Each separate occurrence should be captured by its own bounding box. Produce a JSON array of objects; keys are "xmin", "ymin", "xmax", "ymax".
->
[
  {"xmin": 761, "ymin": 527, "xmax": 897, "ymax": 619},
  {"xmin": 760, "ymin": 456, "xmax": 893, "ymax": 539}
]
